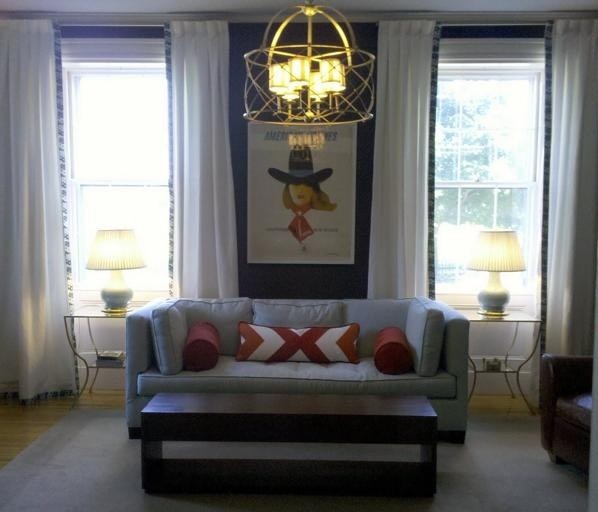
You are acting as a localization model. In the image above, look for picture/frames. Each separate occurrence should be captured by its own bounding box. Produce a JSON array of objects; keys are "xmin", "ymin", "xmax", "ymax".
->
[{"xmin": 244, "ymin": 121, "xmax": 359, "ymax": 267}]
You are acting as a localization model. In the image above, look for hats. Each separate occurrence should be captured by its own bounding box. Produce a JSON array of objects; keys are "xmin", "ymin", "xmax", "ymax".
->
[{"xmin": 268, "ymin": 142, "xmax": 333, "ymax": 184}]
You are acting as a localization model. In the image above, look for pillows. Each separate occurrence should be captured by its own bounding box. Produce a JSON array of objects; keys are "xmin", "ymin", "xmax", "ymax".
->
[
  {"xmin": 372, "ymin": 326, "xmax": 412, "ymax": 375},
  {"xmin": 252, "ymin": 298, "xmax": 344, "ymax": 332},
  {"xmin": 231, "ymin": 319, "xmax": 362, "ymax": 365},
  {"xmin": 149, "ymin": 299, "xmax": 192, "ymax": 378},
  {"xmin": 183, "ymin": 321, "xmax": 220, "ymax": 371},
  {"xmin": 405, "ymin": 297, "xmax": 445, "ymax": 377},
  {"xmin": 176, "ymin": 297, "xmax": 254, "ymax": 357}
]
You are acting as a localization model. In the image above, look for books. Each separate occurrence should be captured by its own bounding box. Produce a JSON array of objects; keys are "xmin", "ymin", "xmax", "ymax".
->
[{"xmin": 95, "ymin": 350, "xmax": 124, "ymax": 368}]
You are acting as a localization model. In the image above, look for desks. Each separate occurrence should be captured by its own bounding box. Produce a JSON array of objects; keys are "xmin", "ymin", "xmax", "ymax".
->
[
  {"xmin": 455, "ymin": 309, "xmax": 544, "ymax": 419},
  {"xmin": 63, "ymin": 300, "xmax": 143, "ymax": 416}
]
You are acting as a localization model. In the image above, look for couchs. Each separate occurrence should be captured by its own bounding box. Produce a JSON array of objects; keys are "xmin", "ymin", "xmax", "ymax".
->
[
  {"xmin": 121, "ymin": 294, "xmax": 472, "ymax": 447},
  {"xmin": 537, "ymin": 352, "xmax": 594, "ymax": 478}
]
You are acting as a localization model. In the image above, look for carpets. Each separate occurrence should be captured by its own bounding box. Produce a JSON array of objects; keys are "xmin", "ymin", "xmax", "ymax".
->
[{"xmin": 0, "ymin": 404, "xmax": 592, "ymax": 512}]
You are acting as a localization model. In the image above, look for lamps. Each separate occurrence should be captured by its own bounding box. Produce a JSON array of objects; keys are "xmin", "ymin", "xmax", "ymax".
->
[
  {"xmin": 240, "ymin": 0, "xmax": 376, "ymax": 126},
  {"xmin": 83, "ymin": 228, "xmax": 147, "ymax": 316},
  {"xmin": 465, "ymin": 229, "xmax": 527, "ymax": 317}
]
